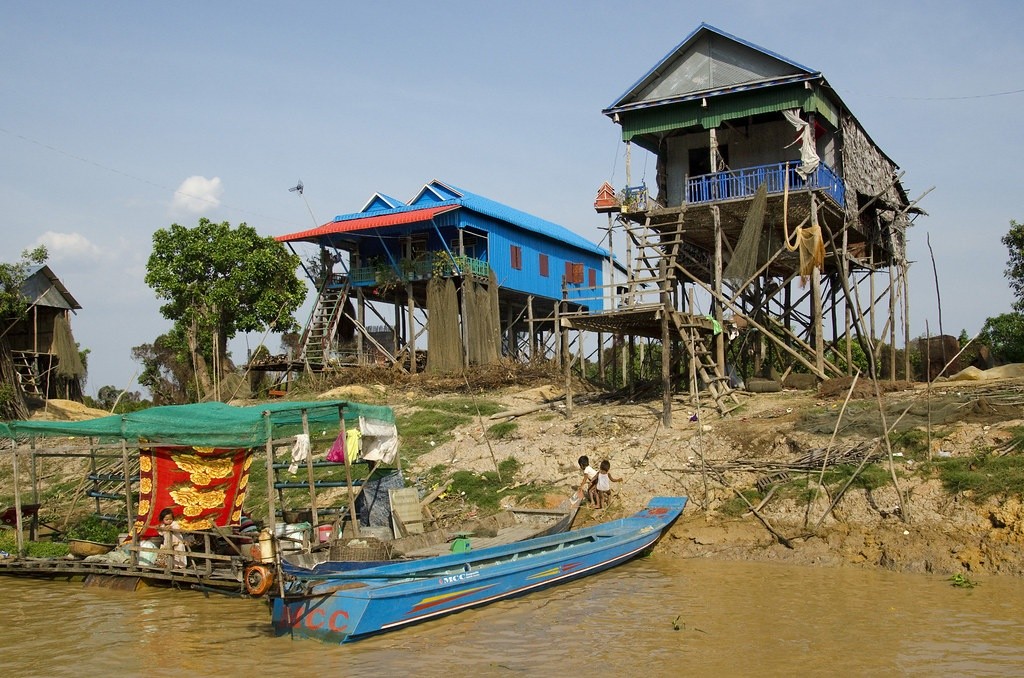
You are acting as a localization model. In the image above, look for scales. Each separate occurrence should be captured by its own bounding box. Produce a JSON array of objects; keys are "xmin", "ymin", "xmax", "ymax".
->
[{"xmin": 449, "ymin": 529, "xmax": 476, "ymax": 553}]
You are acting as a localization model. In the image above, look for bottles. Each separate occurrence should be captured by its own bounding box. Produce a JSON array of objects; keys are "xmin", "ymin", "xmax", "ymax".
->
[{"xmin": 259, "ymin": 528, "xmax": 276, "ymax": 563}]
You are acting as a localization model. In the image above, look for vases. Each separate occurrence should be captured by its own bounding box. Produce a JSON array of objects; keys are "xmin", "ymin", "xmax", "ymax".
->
[{"xmin": 444, "ymin": 270, "xmax": 451, "ymax": 276}]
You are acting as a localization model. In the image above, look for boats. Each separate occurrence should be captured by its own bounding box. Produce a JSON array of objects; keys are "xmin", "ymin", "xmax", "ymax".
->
[
  {"xmin": 271, "ymin": 496, "xmax": 690, "ymax": 646},
  {"xmin": 280, "ymin": 490, "xmax": 584, "ymax": 575},
  {"xmin": 0, "ymin": 399, "xmax": 403, "ymax": 597}
]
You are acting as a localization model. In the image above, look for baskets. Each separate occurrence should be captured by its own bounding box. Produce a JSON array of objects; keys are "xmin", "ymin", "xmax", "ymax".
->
[
  {"xmin": 330, "ymin": 537, "xmax": 395, "ymax": 563},
  {"xmin": 68, "ymin": 538, "xmax": 117, "ymax": 557}
]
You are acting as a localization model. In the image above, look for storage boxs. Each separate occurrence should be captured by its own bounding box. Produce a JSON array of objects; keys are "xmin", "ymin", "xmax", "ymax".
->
[{"xmin": 241, "ymin": 511, "xmax": 392, "ymax": 565}]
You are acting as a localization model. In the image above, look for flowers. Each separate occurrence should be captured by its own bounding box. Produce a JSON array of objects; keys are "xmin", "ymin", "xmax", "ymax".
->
[
  {"xmin": 373, "ymin": 286, "xmax": 382, "ymax": 295},
  {"xmin": 433, "ymin": 250, "xmax": 453, "ymax": 278}
]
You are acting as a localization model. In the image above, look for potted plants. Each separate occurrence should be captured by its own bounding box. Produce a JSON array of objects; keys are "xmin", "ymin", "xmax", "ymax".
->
[{"xmin": 370, "ymin": 249, "xmax": 433, "ymax": 283}]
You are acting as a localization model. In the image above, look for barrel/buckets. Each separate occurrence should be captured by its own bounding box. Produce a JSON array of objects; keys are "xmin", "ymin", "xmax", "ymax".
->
[
  {"xmin": 286, "ymin": 525, "xmax": 304, "ymax": 552},
  {"xmin": 275, "ymin": 522, "xmax": 286, "ymax": 537},
  {"xmin": 118, "ymin": 533, "xmax": 128, "ymax": 545}
]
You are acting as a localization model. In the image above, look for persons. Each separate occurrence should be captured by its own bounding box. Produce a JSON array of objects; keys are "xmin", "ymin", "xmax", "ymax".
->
[
  {"xmin": 590, "ymin": 461, "xmax": 623, "ymax": 509},
  {"xmin": 156, "ymin": 508, "xmax": 187, "ymax": 568},
  {"xmin": 578, "ymin": 456, "xmax": 600, "ymax": 508}
]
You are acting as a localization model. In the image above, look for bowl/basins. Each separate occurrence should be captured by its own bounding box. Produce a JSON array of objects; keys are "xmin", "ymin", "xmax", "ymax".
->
[{"xmin": 67, "ymin": 539, "xmax": 116, "ymax": 556}]
